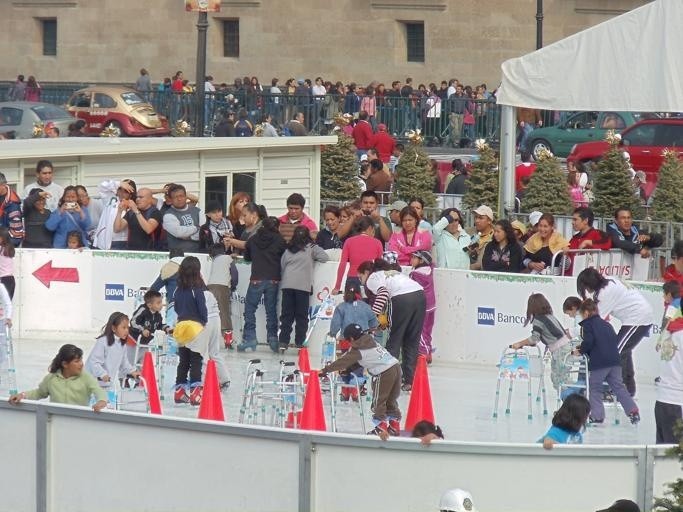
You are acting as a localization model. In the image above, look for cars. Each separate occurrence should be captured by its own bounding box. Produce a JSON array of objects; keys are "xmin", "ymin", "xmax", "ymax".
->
[
  {"xmin": 57, "ymin": 86, "xmax": 171, "ymax": 137},
  {"xmin": 524, "ymin": 109, "xmax": 662, "ymax": 162},
  {"xmin": 0, "ymin": 101, "xmax": 87, "ymax": 139}
]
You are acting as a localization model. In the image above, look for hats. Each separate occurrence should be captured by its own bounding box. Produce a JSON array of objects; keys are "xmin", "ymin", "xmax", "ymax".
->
[
  {"xmin": 224, "ymin": 110, "xmax": 236, "ymax": 116},
  {"xmin": 381, "ymin": 250, "xmax": 398, "ymax": 265},
  {"xmin": 293, "ymin": 225, "xmax": 310, "ymax": 244},
  {"xmin": 439, "ymin": 489, "xmax": 476, "ymax": 512},
  {"xmin": 472, "ymin": 205, "xmax": 493, "ymax": 221},
  {"xmin": 377, "ymin": 123, "xmax": 385, "ymax": 129},
  {"xmin": 411, "ymin": 250, "xmax": 433, "ymax": 264},
  {"xmin": 511, "ymin": 219, "xmax": 526, "ymax": 235},
  {"xmin": 205, "ymin": 200, "xmax": 221, "ymax": 212},
  {"xmin": 343, "ymin": 113, "xmax": 353, "ymax": 118},
  {"xmin": 225, "ymin": 94, "xmax": 234, "ymax": 99},
  {"xmin": 452, "ymin": 165, "xmax": 465, "ymax": 175},
  {"xmin": 636, "ymin": 170, "xmax": 646, "ymax": 184},
  {"xmin": 529, "ymin": 211, "xmax": 543, "ymax": 226},
  {"xmin": 596, "ymin": 499, "xmax": 641, "ymax": 512},
  {"xmin": 387, "ymin": 201, "xmax": 407, "ymax": 212}
]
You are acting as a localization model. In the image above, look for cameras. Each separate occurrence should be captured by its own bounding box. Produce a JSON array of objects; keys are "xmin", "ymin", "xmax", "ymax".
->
[
  {"xmin": 120, "ymin": 199, "xmax": 129, "ymax": 209},
  {"xmin": 360, "ymin": 208, "xmax": 373, "ymax": 215}
]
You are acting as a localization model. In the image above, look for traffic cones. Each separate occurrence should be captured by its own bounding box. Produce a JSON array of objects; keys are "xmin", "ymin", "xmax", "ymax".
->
[
  {"xmin": 132, "ymin": 352, "xmax": 164, "ymax": 414},
  {"xmin": 283, "ymin": 347, "xmax": 436, "ymax": 437},
  {"xmin": 195, "ymin": 355, "xmax": 228, "ymax": 420}
]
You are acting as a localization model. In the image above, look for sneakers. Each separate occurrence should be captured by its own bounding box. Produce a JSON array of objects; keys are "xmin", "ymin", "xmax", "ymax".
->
[
  {"xmin": 589, "ymin": 416, "xmax": 605, "ymax": 425},
  {"xmin": 367, "ymin": 420, "xmax": 400, "ymax": 436},
  {"xmin": 224, "ymin": 331, "xmax": 305, "ymax": 351},
  {"xmin": 173, "ymin": 380, "xmax": 230, "ymax": 405},
  {"xmin": 331, "ymin": 354, "xmax": 432, "ymax": 401},
  {"xmin": 630, "ymin": 411, "xmax": 639, "ymax": 424}
]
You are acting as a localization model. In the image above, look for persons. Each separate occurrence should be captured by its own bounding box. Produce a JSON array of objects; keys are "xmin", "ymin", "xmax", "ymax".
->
[
  {"xmin": 573, "ymin": 298, "xmax": 642, "ymax": 426},
  {"xmin": 518, "ymin": 121, "xmax": 534, "ymax": 137},
  {"xmin": 444, "ymin": 159, "xmax": 472, "ymax": 195},
  {"xmin": 649, "ymin": 297, "xmax": 683, "ymax": 444},
  {"xmin": 7, "ymin": 75, "xmax": 41, "ymax": 102},
  {"xmin": 534, "ymin": 394, "xmax": 593, "ymax": 451},
  {"xmin": 48, "ymin": 120, "xmax": 85, "ymax": 138},
  {"xmin": 516, "ymin": 152, "xmax": 648, "ymax": 212},
  {"xmin": 6, "ymin": 344, "xmax": 110, "ymax": 414},
  {"xmin": 136, "ymin": 68, "xmax": 499, "ymax": 138},
  {"xmin": 511, "ymin": 267, "xmax": 652, "ymax": 402},
  {"xmin": 663, "ymin": 240, "xmax": 683, "ymax": 322},
  {"xmin": 378, "ymin": 420, "xmax": 445, "ymax": 447},
  {"xmin": 316, "ymin": 323, "xmax": 404, "ymax": 437},
  {"xmin": 430, "ymin": 205, "xmax": 664, "ymax": 278},
  {"xmin": 0, "ymin": 160, "xmax": 433, "ymax": 408},
  {"xmin": 343, "ymin": 111, "xmax": 405, "ymax": 173}
]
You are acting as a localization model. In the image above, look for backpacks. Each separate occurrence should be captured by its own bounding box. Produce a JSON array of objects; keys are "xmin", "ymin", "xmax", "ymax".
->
[
  {"xmin": 278, "ymin": 123, "xmax": 291, "ymax": 136},
  {"xmin": 237, "ymin": 124, "xmax": 250, "ymax": 136}
]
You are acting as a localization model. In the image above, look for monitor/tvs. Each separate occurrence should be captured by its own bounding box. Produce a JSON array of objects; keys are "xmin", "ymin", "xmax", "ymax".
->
[{"xmin": 67, "ymin": 202, "xmax": 76, "ymax": 209}]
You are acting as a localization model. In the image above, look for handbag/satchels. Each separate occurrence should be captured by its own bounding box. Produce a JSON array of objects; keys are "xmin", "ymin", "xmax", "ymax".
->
[
  {"xmin": 418, "ymin": 107, "xmax": 431, "ymax": 121},
  {"xmin": 464, "ymin": 112, "xmax": 475, "ymax": 124},
  {"xmin": 319, "ymin": 108, "xmax": 328, "ymax": 119}
]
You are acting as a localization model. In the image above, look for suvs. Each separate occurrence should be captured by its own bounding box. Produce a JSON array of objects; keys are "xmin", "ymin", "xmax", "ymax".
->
[{"xmin": 566, "ymin": 117, "xmax": 682, "ymax": 192}]
